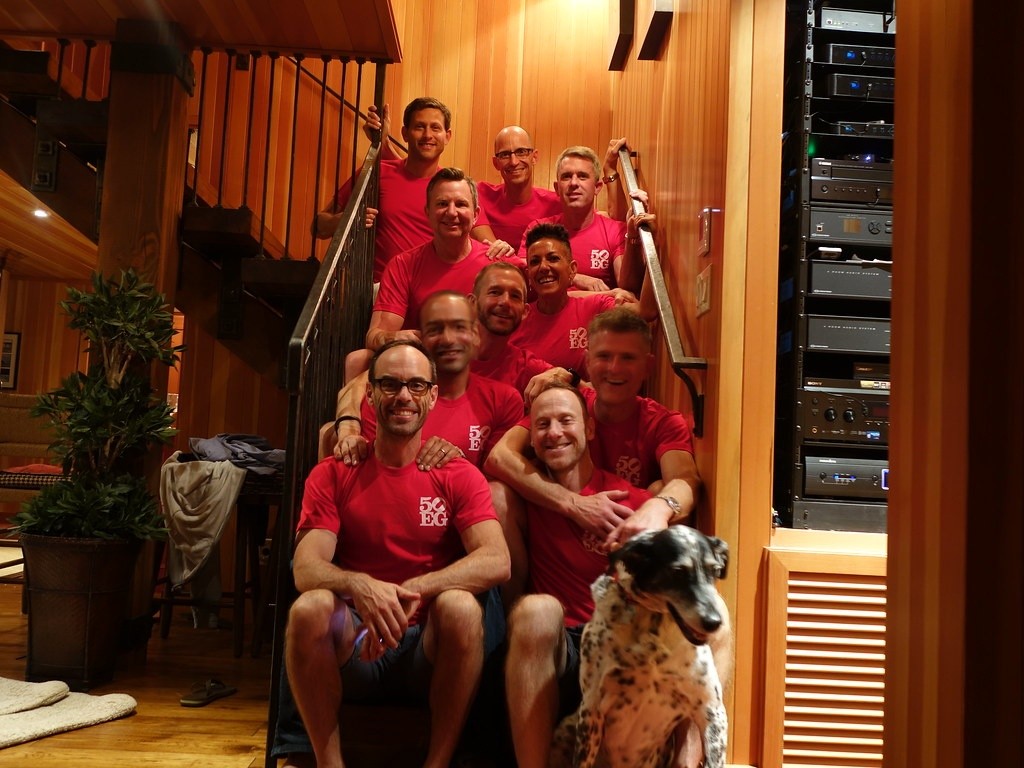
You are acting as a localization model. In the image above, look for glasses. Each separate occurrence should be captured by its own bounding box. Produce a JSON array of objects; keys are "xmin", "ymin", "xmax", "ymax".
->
[
  {"xmin": 496, "ymin": 148, "xmax": 533, "ymax": 158},
  {"xmin": 370, "ymin": 378, "xmax": 433, "ymax": 392}
]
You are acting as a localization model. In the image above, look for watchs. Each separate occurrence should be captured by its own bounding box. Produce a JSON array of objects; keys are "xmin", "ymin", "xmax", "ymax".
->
[{"xmin": 652, "ymin": 494, "xmax": 683, "ymax": 515}]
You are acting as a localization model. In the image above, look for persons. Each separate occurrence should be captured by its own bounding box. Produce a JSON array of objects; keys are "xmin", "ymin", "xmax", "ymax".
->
[
  {"xmin": 363, "ymin": 103, "xmax": 632, "ymax": 255},
  {"xmin": 505, "ymin": 387, "xmax": 706, "ymax": 768},
  {"xmin": 310, "ymin": 98, "xmax": 515, "ymax": 259},
  {"xmin": 333, "ymin": 262, "xmax": 588, "ymax": 466},
  {"xmin": 346, "ymin": 167, "xmax": 526, "ymax": 385},
  {"xmin": 283, "ymin": 339, "xmax": 512, "ymax": 768},
  {"xmin": 361, "ymin": 289, "xmax": 521, "ymax": 585},
  {"xmin": 510, "ymin": 213, "xmax": 659, "ymax": 373},
  {"xmin": 517, "ymin": 145, "xmax": 650, "ymax": 300}
]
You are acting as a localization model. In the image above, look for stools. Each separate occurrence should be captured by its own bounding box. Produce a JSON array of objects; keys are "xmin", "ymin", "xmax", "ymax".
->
[{"xmin": 161, "ymin": 470, "xmax": 271, "ymax": 660}]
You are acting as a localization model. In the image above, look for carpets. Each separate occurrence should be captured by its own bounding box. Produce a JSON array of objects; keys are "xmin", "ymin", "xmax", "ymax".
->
[{"xmin": 0, "ymin": 675, "xmax": 137, "ymax": 746}]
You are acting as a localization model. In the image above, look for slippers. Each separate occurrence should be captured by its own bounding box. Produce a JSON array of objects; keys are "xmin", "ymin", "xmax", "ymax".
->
[{"xmin": 180, "ymin": 679, "xmax": 238, "ymax": 705}]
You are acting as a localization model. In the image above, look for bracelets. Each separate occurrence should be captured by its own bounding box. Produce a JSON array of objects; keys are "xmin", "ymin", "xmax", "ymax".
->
[
  {"xmin": 567, "ymin": 367, "xmax": 582, "ymax": 386},
  {"xmin": 454, "ymin": 445, "xmax": 467, "ymax": 459},
  {"xmin": 601, "ymin": 172, "xmax": 621, "ymax": 183},
  {"xmin": 624, "ymin": 232, "xmax": 642, "ymax": 247},
  {"xmin": 334, "ymin": 416, "xmax": 361, "ymax": 437}
]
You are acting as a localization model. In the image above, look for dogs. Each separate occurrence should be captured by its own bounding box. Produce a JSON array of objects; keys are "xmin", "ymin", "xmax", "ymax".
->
[{"xmin": 546, "ymin": 524, "xmax": 731, "ymax": 768}]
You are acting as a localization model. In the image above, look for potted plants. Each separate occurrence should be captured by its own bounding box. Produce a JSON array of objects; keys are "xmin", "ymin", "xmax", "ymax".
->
[{"xmin": 6, "ymin": 265, "xmax": 189, "ymax": 694}]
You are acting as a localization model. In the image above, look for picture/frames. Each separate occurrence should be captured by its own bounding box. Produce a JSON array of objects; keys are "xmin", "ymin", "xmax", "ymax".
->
[{"xmin": 0, "ymin": 329, "xmax": 20, "ymax": 388}]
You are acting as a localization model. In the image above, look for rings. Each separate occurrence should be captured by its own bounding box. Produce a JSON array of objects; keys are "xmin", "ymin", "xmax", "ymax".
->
[{"xmin": 438, "ymin": 448, "xmax": 447, "ymax": 455}]
